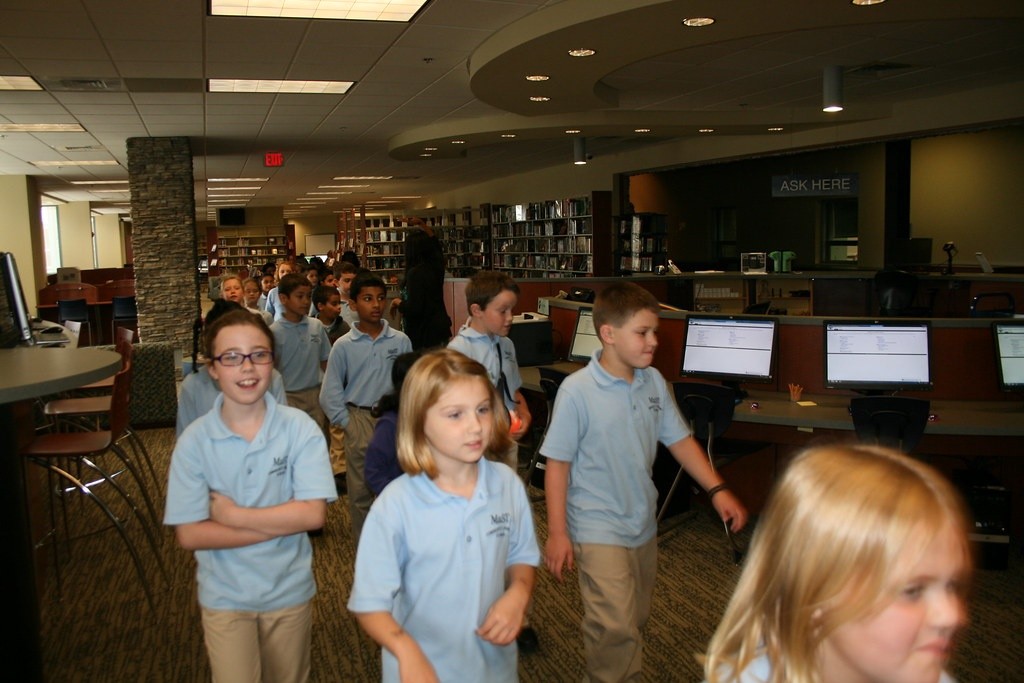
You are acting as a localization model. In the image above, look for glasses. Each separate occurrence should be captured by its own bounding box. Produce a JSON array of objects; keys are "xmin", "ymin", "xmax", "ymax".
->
[
  {"xmin": 244, "ymin": 289, "xmax": 261, "ymax": 294},
  {"xmin": 211, "ymin": 351, "xmax": 276, "ymax": 367}
]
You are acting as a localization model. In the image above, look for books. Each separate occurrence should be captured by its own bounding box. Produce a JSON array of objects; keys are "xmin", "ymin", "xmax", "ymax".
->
[
  {"xmin": 218, "ymin": 238, "xmax": 286, "ymax": 265},
  {"xmin": 364, "ymin": 222, "xmax": 390, "ymax": 269},
  {"xmin": 493, "ymin": 195, "xmax": 592, "ymax": 278},
  {"xmin": 621, "ymin": 236, "xmax": 668, "ymax": 272},
  {"xmin": 391, "ymin": 222, "xmax": 490, "ymax": 269}
]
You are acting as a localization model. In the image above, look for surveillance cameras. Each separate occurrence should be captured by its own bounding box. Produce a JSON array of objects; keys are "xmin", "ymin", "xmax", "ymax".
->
[{"xmin": 586, "ymin": 155, "xmax": 593, "ymax": 160}]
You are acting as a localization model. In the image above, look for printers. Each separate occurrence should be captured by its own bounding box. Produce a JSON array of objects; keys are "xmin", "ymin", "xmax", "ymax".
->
[{"xmin": 507, "ymin": 312, "xmax": 554, "ymax": 367}]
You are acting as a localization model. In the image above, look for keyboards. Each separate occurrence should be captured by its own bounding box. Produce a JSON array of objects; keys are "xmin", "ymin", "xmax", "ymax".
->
[{"xmin": 34, "ymin": 334, "xmax": 71, "ymax": 345}]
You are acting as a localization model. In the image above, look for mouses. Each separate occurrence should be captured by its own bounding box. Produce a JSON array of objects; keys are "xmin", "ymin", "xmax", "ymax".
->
[
  {"xmin": 751, "ymin": 401, "xmax": 759, "ymax": 410},
  {"xmin": 40, "ymin": 326, "xmax": 63, "ymax": 334},
  {"xmin": 928, "ymin": 414, "xmax": 938, "ymax": 421}
]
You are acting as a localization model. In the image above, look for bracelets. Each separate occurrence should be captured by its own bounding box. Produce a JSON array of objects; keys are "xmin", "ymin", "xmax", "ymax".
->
[{"xmin": 707, "ymin": 482, "xmax": 731, "ymax": 499}]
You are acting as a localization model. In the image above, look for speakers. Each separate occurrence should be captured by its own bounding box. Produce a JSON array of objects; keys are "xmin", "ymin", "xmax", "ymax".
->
[{"xmin": 530, "ymin": 425, "xmax": 549, "ymax": 490}]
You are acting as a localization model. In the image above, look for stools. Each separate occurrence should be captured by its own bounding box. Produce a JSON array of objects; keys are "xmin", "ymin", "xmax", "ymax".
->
[{"xmin": 23, "ymin": 326, "xmax": 159, "ymax": 621}]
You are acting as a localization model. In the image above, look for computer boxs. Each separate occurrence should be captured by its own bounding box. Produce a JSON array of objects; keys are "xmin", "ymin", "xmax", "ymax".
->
[
  {"xmin": 652, "ymin": 444, "xmax": 691, "ymax": 520},
  {"xmin": 953, "ymin": 468, "xmax": 1011, "ymax": 571}
]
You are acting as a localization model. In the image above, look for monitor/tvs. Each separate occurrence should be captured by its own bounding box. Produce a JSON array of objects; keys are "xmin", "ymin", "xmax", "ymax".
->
[
  {"xmin": 992, "ymin": 319, "xmax": 1024, "ymax": 392},
  {"xmin": 2, "ymin": 253, "xmax": 31, "ymax": 341},
  {"xmin": 679, "ymin": 315, "xmax": 779, "ymax": 401},
  {"xmin": 219, "ymin": 208, "xmax": 245, "ymax": 225},
  {"xmin": 822, "ymin": 319, "xmax": 934, "ymax": 398},
  {"xmin": 568, "ymin": 307, "xmax": 603, "ymax": 365},
  {"xmin": 907, "ymin": 238, "xmax": 932, "ymax": 267}
]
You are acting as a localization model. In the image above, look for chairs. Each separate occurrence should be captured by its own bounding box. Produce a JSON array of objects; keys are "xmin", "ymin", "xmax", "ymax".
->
[
  {"xmin": 57, "ymin": 298, "xmax": 96, "ymax": 346},
  {"xmin": 525, "ymin": 376, "xmax": 563, "ymax": 494},
  {"xmin": 112, "ymin": 295, "xmax": 138, "ymax": 342},
  {"xmin": 656, "ymin": 381, "xmax": 778, "ymax": 561},
  {"xmin": 851, "ymin": 397, "xmax": 931, "ymax": 453}
]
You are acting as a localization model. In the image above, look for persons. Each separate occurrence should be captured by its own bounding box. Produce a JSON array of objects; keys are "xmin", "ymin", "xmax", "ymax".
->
[
  {"xmin": 446, "ymin": 272, "xmax": 531, "ymax": 475},
  {"xmin": 296, "ymin": 253, "xmax": 363, "ymax": 328},
  {"xmin": 267, "ymin": 274, "xmax": 333, "ymax": 432},
  {"xmin": 706, "ymin": 442, "xmax": 974, "ymax": 683},
  {"xmin": 267, "ymin": 261, "xmax": 296, "ymax": 313},
  {"xmin": 390, "ymin": 216, "xmax": 452, "ymax": 351},
  {"xmin": 538, "ymin": 283, "xmax": 748, "ymax": 683},
  {"xmin": 176, "ymin": 299, "xmax": 287, "ymax": 439},
  {"xmin": 365, "ymin": 352, "xmax": 423, "ymax": 498},
  {"xmin": 346, "ymin": 350, "xmax": 541, "ymax": 683},
  {"xmin": 318, "ymin": 274, "xmax": 420, "ymax": 550},
  {"xmin": 216, "ymin": 266, "xmax": 275, "ymax": 318},
  {"xmin": 313, "ymin": 284, "xmax": 351, "ymax": 346},
  {"xmin": 163, "ymin": 311, "xmax": 338, "ymax": 682}
]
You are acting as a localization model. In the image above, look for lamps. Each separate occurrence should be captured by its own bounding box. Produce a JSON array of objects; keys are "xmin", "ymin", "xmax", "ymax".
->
[
  {"xmin": 575, "ymin": 136, "xmax": 588, "ymax": 165},
  {"xmin": 821, "ymin": 66, "xmax": 844, "ymax": 112}
]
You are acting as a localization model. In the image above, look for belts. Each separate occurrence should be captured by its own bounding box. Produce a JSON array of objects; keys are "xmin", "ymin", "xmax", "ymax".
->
[{"xmin": 347, "ymin": 403, "xmax": 375, "ymax": 411}]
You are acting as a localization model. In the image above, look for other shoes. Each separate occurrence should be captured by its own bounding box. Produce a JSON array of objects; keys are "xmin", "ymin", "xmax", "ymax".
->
[
  {"xmin": 340, "ymin": 475, "xmax": 347, "ymax": 492},
  {"xmin": 516, "ymin": 620, "xmax": 537, "ymax": 646}
]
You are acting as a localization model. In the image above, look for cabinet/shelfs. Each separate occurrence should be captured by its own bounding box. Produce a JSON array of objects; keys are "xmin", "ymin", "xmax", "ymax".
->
[
  {"xmin": 220, "ymin": 225, "xmax": 294, "ymax": 270},
  {"xmin": 198, "ymin": 229, "xmax": 208, "ymax": 255},
  {"xmin": 334, "ymin": 190, "xmax": 668, "ymax": 291}
]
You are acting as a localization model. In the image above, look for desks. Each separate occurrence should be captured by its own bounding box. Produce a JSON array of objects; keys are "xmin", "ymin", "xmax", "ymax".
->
[
  {"xmin": 36, "ymin": 299, "xmax": 111, "ymax": 343},
  {"xmin": 0, "ymin": 344, "xmax": 123, "ymax": 401}
]
